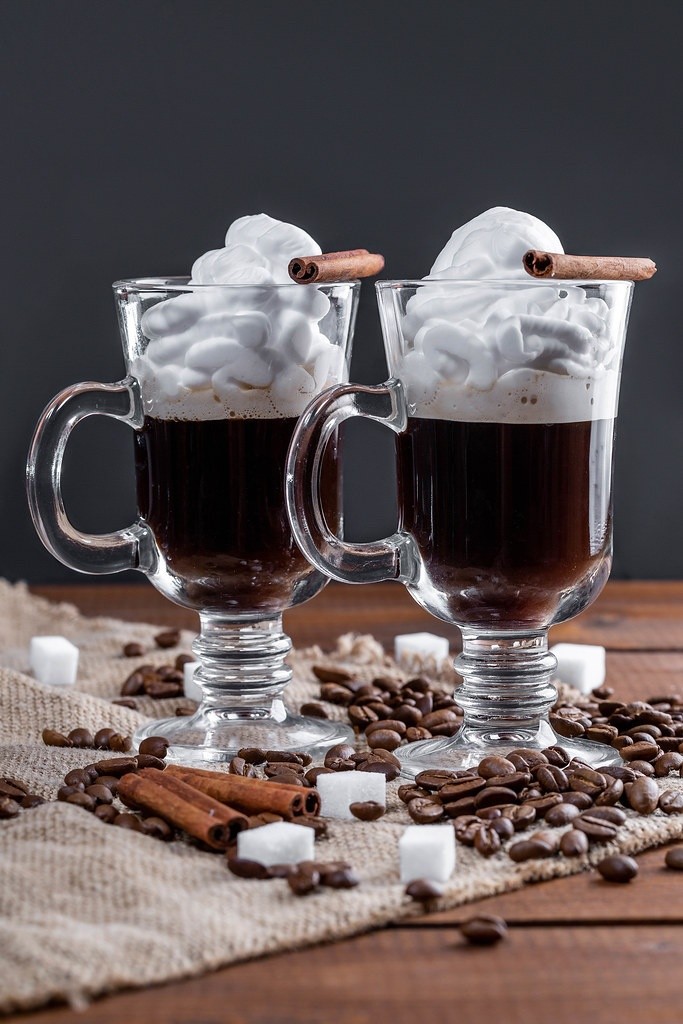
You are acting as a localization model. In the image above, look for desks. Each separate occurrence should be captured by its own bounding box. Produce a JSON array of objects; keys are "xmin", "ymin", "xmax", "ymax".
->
[{"xmin": 0, "ymin": 580, "xmax": 683, "ymax": 1024}]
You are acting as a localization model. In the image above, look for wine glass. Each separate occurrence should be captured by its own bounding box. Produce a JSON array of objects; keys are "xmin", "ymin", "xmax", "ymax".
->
[{"xmin": 27, "ymin": 275, "xmax": 636, "ymax": 784}]
